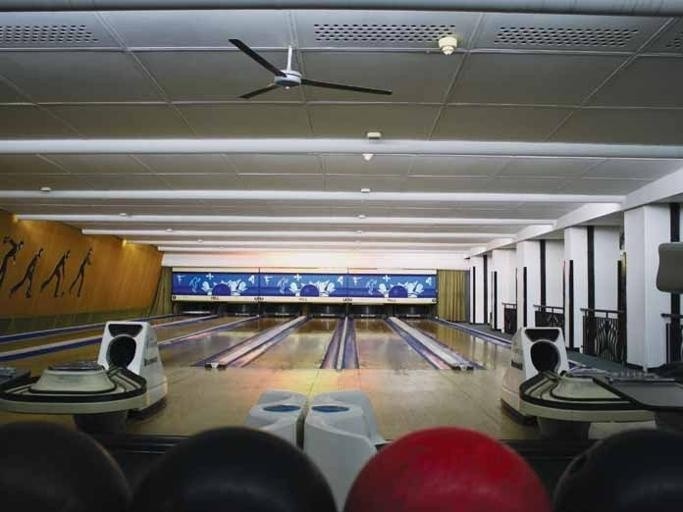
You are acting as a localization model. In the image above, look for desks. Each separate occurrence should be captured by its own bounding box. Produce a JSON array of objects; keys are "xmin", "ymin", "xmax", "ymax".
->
[{"xmin": 0, "ymin": 420, "xmax": 682, "ymax": 512}]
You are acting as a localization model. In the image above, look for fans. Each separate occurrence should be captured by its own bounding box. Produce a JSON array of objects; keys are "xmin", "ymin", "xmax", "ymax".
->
[{"xmin": 228, "ymin": 38, "xmax": 392, "ymax": 99}]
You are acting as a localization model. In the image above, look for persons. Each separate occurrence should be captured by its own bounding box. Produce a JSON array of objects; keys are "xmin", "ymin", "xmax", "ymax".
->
[
  {"xmin": 64, "ymin": 248, "xmax": 96, "ymax": 296},
  {"xmin": 0, "ymin": 234, "xmax": 24, "ymax": 286},
  {"xmin": 7, "ymin": 247, "xmax": 43, "ymax": 297},
  {"xmin": 39, "ymin": 250, "xmax": 71, "ymax": 300}
]
